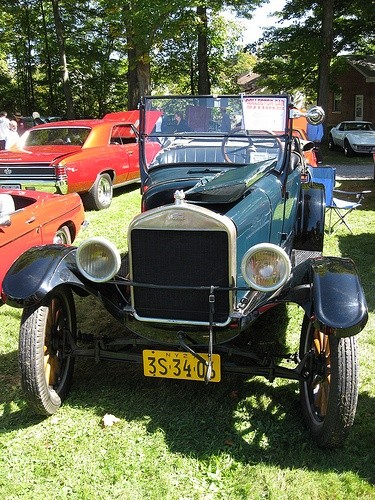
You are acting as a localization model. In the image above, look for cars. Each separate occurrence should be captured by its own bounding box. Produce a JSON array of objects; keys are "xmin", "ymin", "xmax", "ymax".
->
[
  {"xmin": 20, "ymin": 96, "xmax": 369, "ymax": 452},
  {"xmin": 0, "ymin": 109, "xmax": 162, "ymax": 212},
  {"xmin": 0, "ymin": 188, "xmax": 89, "ymax": 302},
  {"xmin": 209, "ymin": 119, "xmax": 220, "ymax": 132},
  {"xmin": 328, "ymin": 121, "xmax": 375, "ymax": 157},
  {"xmin": 160, "ymin": 114, "xmax": 179, "ymax": 133}
]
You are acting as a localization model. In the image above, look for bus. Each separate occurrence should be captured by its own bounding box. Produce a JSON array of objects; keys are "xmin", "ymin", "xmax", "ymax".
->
[{"xmin": 16, "ymin": 116, "xmax": 60, "ymax": 135}]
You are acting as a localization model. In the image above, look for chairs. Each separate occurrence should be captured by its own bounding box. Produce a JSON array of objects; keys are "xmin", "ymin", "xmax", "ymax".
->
[
  {"xmin": 0, "ymin": 195, "xmax": 15, "ymax": 216},
  {"xmin": 307, "ymin": 167, "xmax": 371, "ymax": 238}
]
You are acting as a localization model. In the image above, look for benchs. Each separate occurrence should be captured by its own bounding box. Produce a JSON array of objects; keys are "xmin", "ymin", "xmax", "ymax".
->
[{"xmin": 157, "ymin": 146, "xmax": 280, "ymax": 165}]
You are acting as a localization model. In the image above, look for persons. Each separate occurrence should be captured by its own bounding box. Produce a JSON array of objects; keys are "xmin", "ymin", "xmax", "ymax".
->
[
  {"xmin": 0, "ymin": 107, "xmax": 230, "ymax": 150},
  {"xmin": 307, "ymin": 122, "xmax": 323, "ymax": 163}
]
